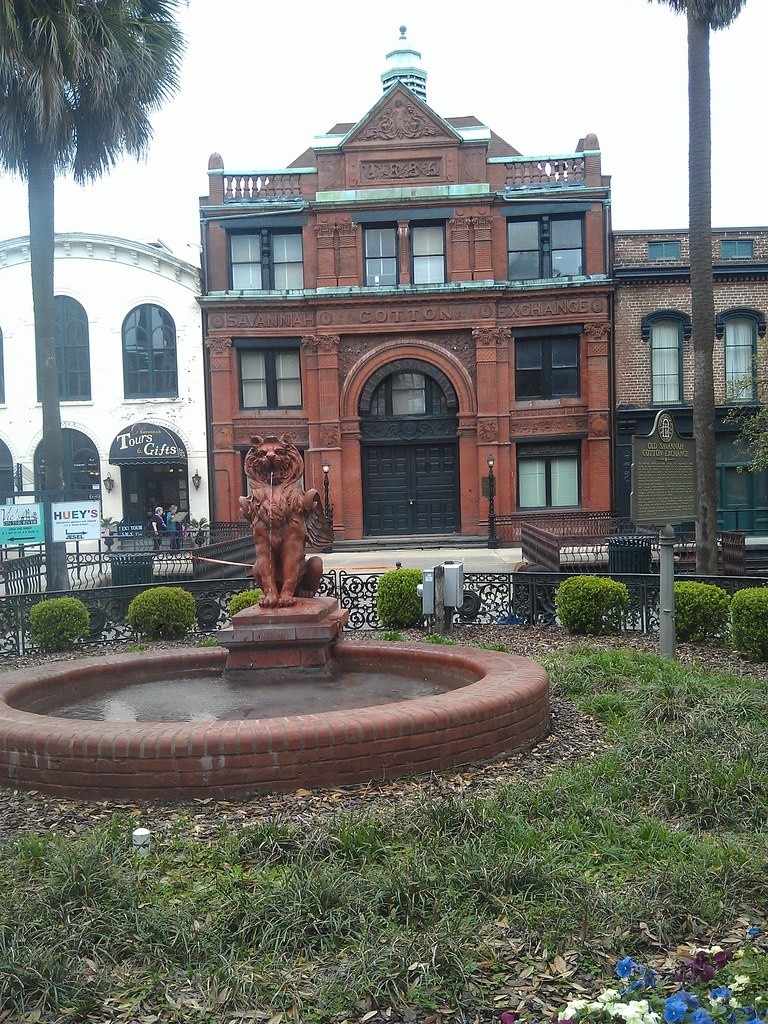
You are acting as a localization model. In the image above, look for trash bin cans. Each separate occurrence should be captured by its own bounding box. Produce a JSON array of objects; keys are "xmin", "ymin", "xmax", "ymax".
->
[
  {"xmin": 106, "ymin": 554, "xmax": 154, "ymax": 618},
  {"xmin": 605, "ymin": 536, "xmax": 656, "ymax": 605}
]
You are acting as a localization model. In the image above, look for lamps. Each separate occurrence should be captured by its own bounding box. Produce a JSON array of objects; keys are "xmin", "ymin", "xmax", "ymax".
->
[
  {"xmin": 191, "ymin": 469, "xmax": 202, "ymax": 491},
  {"xmin": 103, "ymin": 472, "xmax": 114, "ymax": 493}
]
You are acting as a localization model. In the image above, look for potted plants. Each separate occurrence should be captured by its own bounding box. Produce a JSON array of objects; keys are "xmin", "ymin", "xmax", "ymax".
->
[
  {"xmin": 101, "ymin": 517, "xmax": 120, "ymax": 552},
  {"xmin": 190, "ymin": 519, "xmax": 211, "ymax": 548}
]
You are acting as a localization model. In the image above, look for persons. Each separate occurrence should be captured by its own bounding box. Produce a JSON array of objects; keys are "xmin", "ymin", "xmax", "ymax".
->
[
  {"xmin": 167, "ymin": 505, "xmax": 183, "ymax": 549},
  {"xmin": 150, "ymin": 507, "xmax": 167, "ymax": 550}
]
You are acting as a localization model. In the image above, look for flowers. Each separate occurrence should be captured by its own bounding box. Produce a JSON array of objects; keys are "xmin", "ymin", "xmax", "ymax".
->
[{"xmin": 496, "ymin": 926, "xmax": 768, "ymax": 1024}]
